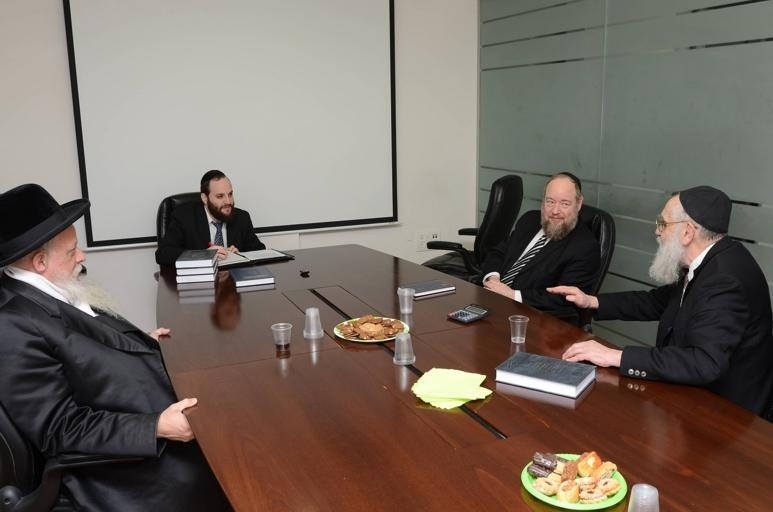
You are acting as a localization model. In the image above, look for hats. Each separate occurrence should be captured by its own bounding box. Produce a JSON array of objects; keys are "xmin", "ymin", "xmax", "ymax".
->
[
  {"xmin": 680, "ymin": 186, "xmax": 731, "ymax": 233},
  {"xmin": 0, "ymin": 184, "xmax": 90, "ymax": 267}
]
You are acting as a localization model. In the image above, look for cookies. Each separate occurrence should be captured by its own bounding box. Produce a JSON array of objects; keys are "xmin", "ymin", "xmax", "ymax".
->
[{"xmin": 336, "ymin": 314, "xmax": 404, "ymax": 341}]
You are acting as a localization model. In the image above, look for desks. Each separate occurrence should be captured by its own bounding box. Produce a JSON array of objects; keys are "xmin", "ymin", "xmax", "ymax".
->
[{"xmin": 152, "ymin": 244, "xmax": 771, "ymax": 512}]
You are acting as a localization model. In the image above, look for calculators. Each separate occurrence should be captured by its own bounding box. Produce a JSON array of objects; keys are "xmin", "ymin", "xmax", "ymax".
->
[{"xmin": 446, "ymin": 303, "xmax": 489, "ymax": 323}]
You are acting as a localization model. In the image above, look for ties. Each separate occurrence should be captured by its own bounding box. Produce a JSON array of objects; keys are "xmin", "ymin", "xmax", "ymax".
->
[
  {"xmin": 211, "ymin": 221, "xmax": 223, "ymax": 246},
  {"xmin": 501, "ymin": 235, "xmax": 546, "ymax": 288}
]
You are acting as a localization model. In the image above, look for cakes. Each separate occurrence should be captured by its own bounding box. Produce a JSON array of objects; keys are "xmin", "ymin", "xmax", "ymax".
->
[{"xmin": 528, "ymin": 450, "xmax": 621, "ymax": 506}]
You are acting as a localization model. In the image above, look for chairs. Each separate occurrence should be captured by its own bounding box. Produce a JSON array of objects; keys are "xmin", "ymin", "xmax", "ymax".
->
[
  {"xmin": 154, "ymin": 192, "xmax": 216, "ymax": 282},
  {"xmin": 421, "ymin": 174, "xmax": 523, "ymax": 287},
  {"xmin": 510, "ymin": 203, "xmax": 615, "ymax": 335},
  {"xmin": 1, "ymin": 408, "xmax": 158, "ymax": 511}
]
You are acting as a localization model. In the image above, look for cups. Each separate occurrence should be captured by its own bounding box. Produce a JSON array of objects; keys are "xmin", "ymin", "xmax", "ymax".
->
[
  {"xmin": 393, "ymin": 332, "xmax": 415, "ymax": 365},
  {"xmin": 398, "ymin": 287, "xmax": 415, "ymax": 314},
  {"xmin": 302, "ymin": 305, "xmax": 325, "ymax": 339},
  {"xmin": 510, "ymin": 341, "xmax": 526, "ymax": 356},
  {"xmin": 273, "ymin": 351, "xmax": 295, "ymax": 377},
  {"xmin": 627, "ymin": 484, "xmax": 661, "ymax": 512},
  {"xmin": 301, "ymin": 337, "xmax": 325, "ymax": 367},
  {"xmin": 270, "ymin": 322, "xmax": 292, "ymax": 355},
  {"xmin": 393, "ymin": 365, "xmax": 411, "ymax": 393},
  {"xmin": 400, "ymin": 312, "xmax": 414, "ymax": 333},
  {"xmin": 509, "ymin": 315, "xmax": 529, "ymax": 345}
]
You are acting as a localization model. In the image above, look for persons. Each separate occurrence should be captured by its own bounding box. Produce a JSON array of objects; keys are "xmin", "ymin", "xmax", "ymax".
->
[
  {"xmin": 0, "ymin": 183, "xmax": 197, "ymax": 512},
  {"xmin": 546, "ymin": 185, "xmax": 772, "ymax": 425},
  {"xmin": 482, "ymin": 172, "xmax": 602, "ymax": 327},
  {"xmin": 210, "ymin": 275, "xmax": 241, "ymax": 332},
  {"xmin": 155, "ymin": 170, "xmax": 266, "ymax": 275}
]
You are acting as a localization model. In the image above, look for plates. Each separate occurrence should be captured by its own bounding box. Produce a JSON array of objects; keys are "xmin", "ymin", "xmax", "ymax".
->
[
  {"xmin": 331, "ymin": 316, "xmax": 410, "ymax": 345},
  {"xmin": 520, "ymin": 486, "xmax": 626, "ymax": 512},
  {"xmin": 333, "ymin": 335, "xmax": 395, "ymax": 352},
  {"xmin": 521, "ymin": 454, "xmax": 629, "ymax": 512}
]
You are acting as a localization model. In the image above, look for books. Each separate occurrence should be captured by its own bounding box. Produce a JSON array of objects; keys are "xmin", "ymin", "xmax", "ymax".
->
[
  {"xmin": 494, "ymin": 351, "xmax": 596, "ymax": 400},
  {"xmin": 177, "ymin": 281, "xmax": 216, "ymax": 304},
  {"xmin": 236, "ymin": 283, "xmax": 276, "ymax": 293},
  {"xmin": 175, "ymin": 249, "xmax": 295, "ymax": 288},
  {"xmin": 413, "ymin": 290, "xmax": 456, "ymax": 301},
  {"xmin": 398, "ymin": 279, "xmax": 456, "ymax": 298},
  {"xmin": 495, "ymin": 381, "xmax": 596, "ymax": 411}
]
